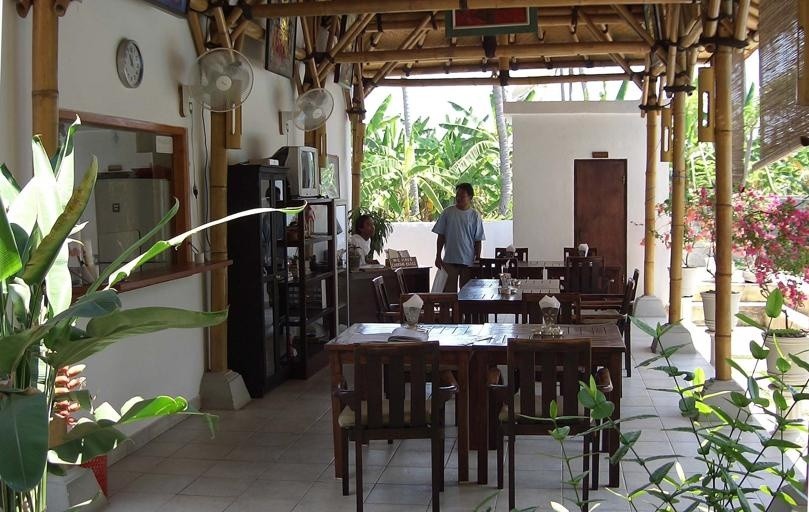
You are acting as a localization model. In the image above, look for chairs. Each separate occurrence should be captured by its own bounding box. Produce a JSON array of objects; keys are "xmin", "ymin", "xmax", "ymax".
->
[{"xmin": 324, "ymin": 246, "xmax": 639, "ymax": 512}]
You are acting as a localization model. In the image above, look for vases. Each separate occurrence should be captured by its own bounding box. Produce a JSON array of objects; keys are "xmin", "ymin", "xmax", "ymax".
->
[
  {"xmin": 669, "ymin": 266, "xmax": 696, "ymax": 297},
  {"xmin": 700, "ymin": 291, "xmax": 739, "ymax": 332},
  {"xmin": 761, "ymin": 329, "xmax": 809, "ymax": 387}
]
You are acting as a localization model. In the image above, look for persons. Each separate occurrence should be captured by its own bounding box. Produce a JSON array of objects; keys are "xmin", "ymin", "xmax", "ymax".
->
[
  {"xmin": 431, "ymin": 182, "xmax": 485, "ymax": 293},
  {"xmin": 347, "ymin": 213, "xmax": 375, "ymax": 269}
]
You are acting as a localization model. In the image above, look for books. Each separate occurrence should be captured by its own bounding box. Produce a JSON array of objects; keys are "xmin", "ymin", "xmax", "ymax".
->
[{"xmin": 349, "ymin": 327, "xmax": 427, "ymax": 346}]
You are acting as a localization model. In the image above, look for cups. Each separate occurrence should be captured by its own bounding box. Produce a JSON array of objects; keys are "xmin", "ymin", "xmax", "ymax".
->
[
  {"xmin": 541, "ymin": 307, "xmax": 559, "ymax": 334},
  {"xmin": 403, "ymin": 304, "xmax": 421, "ymax": 331},
  {"xmin": 500, "ymin": 273, "xmax": 512, "ymax": 291},
  {"xmin": 505, "ymin": 251, "xmax": 514, "ymax": 259}
]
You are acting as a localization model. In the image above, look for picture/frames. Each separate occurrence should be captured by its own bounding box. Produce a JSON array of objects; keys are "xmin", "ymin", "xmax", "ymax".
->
[
  {"xmin": 265, "ymin": 0, "xmax": 298, "ymax": 80},
  {"xmin": 444, "ymin": 6, "xmax": 538, "ymax": 38},
  {"xmin": 334, "ymin": 15, "xmax": 357, "ymax": 89}
]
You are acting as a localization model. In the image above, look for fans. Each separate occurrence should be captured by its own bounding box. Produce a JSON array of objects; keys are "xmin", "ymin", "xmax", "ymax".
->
[
  {"xmin": 278, "ymin": 88, "xmax": 334, "ymax": 135},
  {"xmin": 179, "ymin": 47, "xmax": 254, "ymax": 117}
]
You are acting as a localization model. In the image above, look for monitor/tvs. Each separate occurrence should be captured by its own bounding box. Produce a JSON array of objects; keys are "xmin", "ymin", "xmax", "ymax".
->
[{"xmin": 272, "ymin": 144, "xmax": 322, "ymax": 198}]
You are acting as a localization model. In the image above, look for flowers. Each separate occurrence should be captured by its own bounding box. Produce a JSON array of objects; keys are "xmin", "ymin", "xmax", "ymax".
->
[
  {"xmin": 631, "ymin": 185, "xmax": 705, "ymax": 268},
  {"xmin": 731, "ymin": 183, "xmax": 809, "ymax": 328},
  {"xmin": 685, "ymin": 187, "xmax": 745, "ymax": 279}
]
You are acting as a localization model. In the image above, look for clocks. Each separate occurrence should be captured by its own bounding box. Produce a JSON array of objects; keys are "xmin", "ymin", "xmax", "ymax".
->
[{"xmin": 116, "ymin": 38, "xmax": 143, "ymax": 87}]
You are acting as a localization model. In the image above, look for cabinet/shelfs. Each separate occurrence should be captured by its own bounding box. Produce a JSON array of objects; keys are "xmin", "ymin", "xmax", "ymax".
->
[
  {"xmin": 228, "ymin": 163, "xmax": 289, "ymax": 398},
  {"xmin": 285, "ymin": 198, "xmax": 350, "ymax": 380}
]
